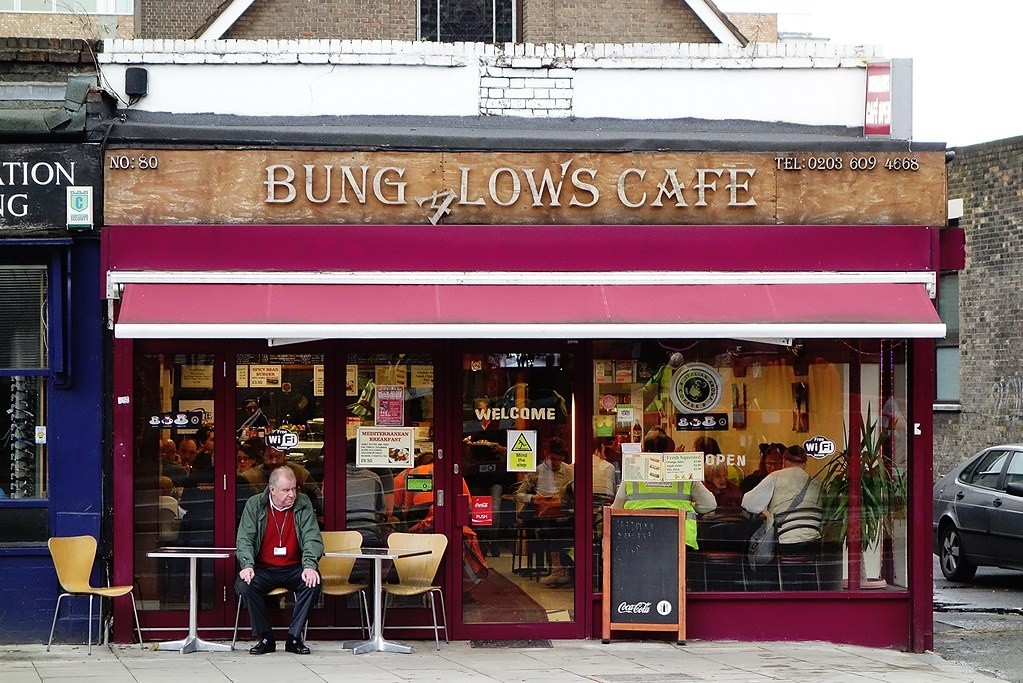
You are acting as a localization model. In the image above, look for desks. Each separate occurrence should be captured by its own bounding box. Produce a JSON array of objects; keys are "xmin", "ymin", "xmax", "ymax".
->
[
  {"xmin": 501, "ymin": 493, "xmax": 546, "ymax": 578},
  {"xmin": 146, "ymin": 547, "xmax": 237, "ymax": 656},
  {"xmin": 322, "ymin": 549, "xmax": 432, "ymax": 655}
]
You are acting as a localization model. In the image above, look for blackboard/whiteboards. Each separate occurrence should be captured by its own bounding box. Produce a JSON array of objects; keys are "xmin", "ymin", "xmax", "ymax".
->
[{"xmin": 603, "ymin": 506, "xmax": 686, "ymax": 631}]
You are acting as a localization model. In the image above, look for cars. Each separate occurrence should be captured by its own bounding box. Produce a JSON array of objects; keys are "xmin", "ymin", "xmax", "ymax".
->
[{"xmin": 933, "ymin": 443, "xmax": 1023, "ymax": 584}]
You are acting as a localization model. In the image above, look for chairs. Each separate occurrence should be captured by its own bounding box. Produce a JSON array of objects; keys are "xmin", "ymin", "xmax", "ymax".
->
[
  {"xmin": 507, "ymin": 491, "xmax": 827, "ymax": 592},
  {"xmin": 48, "ymin": 495, "xmax": 453, "ymax": 657}
]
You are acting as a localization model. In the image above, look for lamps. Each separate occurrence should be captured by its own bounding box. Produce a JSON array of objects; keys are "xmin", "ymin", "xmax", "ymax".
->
[{"xmin": 669, "ymin": 351, "xmax": 684, "ymax": 369}]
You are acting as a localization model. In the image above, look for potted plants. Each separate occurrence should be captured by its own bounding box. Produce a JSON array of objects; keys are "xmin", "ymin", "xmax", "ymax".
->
[{"xmin": 810, "ymin": 400, "xmax": 909, "ymax": 589}]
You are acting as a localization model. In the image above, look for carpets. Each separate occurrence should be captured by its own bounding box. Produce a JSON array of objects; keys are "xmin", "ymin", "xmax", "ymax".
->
[{"xmin": 463, "ymin": 568, "xmax": 554, "ymax": 649}]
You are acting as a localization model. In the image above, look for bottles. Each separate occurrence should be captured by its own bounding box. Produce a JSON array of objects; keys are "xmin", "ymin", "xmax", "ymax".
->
[
  {"xmin": 613, "ymin": 462, "xmax": 620, "ymax": 484},
  {"xmin": 633, "ymin": 419, "xmax": 642, "ymax": 442}
]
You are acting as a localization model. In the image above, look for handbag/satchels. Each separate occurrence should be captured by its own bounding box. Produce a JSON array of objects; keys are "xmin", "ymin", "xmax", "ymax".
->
[{"xmin": 747, "ymin": 523, "xmax": 775, "ymax": 569}]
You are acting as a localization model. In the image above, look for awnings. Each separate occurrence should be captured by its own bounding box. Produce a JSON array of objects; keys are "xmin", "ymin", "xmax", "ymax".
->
[{"xmin": 115, "ymin": 282, "xmax": 947, "ymax": 338}]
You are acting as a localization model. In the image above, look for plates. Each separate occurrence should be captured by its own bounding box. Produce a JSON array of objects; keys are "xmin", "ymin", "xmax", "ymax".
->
[
  {"xmin": 678, "ymin": 421, "xmax": 717, "ymax": 427},
  {"xmin": 149, "ymin": 419, "xmax": 189, "ymax": 424}
]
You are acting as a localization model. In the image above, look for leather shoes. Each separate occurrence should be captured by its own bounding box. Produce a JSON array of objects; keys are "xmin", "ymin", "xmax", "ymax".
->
[
  {"xmin": 284, "ymin": 634, "xmax": 310, "ymax": 654},
  {"xmin": 249, "ymin": 632, "xmax": 276, "ymax": 654}
]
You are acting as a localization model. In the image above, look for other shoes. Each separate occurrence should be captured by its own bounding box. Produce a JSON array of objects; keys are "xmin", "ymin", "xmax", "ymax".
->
[
  {"xmin": 540, "ymin": 570, "xmax": 571, "ymax": 585},
  {"xmin": 464, "ymin": 592, "xmax": 478, "ymax": 603}
]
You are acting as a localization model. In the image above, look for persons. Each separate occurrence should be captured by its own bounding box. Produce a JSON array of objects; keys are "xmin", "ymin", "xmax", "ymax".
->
[
  {"xmin": 347, "ymin": 438, "xmax": 395, "ymax": 585},
  {"xmin": 740, "ymin": 441, "xmax": 824, "ymax": 589},
  {"xmin": 395, "ymin": 452, "xmax": 472, "ymax": 512},
  {"xmin": 463, "ymin": 398, "xmax": 506, "ymax": 556},
  {"xmin": 513, "ymin": 437, "xmax": 617, "ymax": 585},
  {"xmin": 236, "ymin": 466, "xmax": 325, "ymax": 654},
  {"xmin": 611, "ymin": 427, "xmax": 743, "ymax": 592},
  {"xmin": 159, "ymin": 426, "xmax": 323, "ymax": 605}
]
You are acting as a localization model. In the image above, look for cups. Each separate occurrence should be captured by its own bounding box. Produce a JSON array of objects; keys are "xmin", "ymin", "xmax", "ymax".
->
[
  {"xmin": 151, "ymin": 414, "xmax": 187, "ymax": 422},
  {"xmin": 680, "ymin": 416, "xmax": 714, "ymax": 425}
]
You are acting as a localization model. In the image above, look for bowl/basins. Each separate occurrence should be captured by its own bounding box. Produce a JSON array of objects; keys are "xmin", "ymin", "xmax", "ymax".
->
[{"xmin": 470, "ymin": 445, "xmax": 500, "ymax": 461}]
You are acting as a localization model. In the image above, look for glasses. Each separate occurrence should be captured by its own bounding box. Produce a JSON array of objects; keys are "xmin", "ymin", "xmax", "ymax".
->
[
  {"xmin": 764, "ymin": 462, "xmax": 781, "ymax": 467},
  {"xmin": 235, "ymin": 455, "xmax": 250, "ymax": 462}
]
